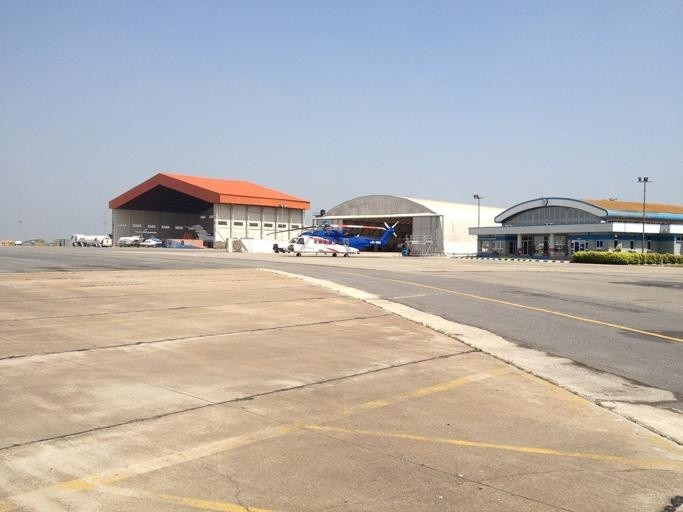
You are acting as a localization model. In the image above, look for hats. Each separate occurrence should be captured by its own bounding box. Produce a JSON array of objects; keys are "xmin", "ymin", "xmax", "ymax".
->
[{"xmin": 139, "ymin": 238, "xmax": 162, "ymax": 247}]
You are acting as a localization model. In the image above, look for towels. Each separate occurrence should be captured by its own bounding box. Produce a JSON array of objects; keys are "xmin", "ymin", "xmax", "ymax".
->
[
  {"xmin": 227, "ymin": 238, "xmax": 233, "ymax": 253},
  {"xmin": 403, "ymin": 248, "xmax": 409, "ymax": 256},
  {"xmin": 50, "ymin": 241, "xmax": 55, "ymax": 247},
  {"xmin": 60, "ymin": 240, "xmax": 66, "ymax": 246}
]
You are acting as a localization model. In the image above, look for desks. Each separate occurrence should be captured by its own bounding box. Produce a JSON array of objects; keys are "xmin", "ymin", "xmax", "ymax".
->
[
  {"xmin": 637, "ymin": 176, "xmax": 649, "ymax": 254},
  {"xmin": 473, "ymin": 193, "xmax": 483, "ymax": 227}
]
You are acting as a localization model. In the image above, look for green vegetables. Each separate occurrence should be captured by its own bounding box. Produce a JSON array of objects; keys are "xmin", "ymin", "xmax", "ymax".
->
[{"xmin": 266, "ymin": 221, "xmax": 400, "ymax": 257}]
